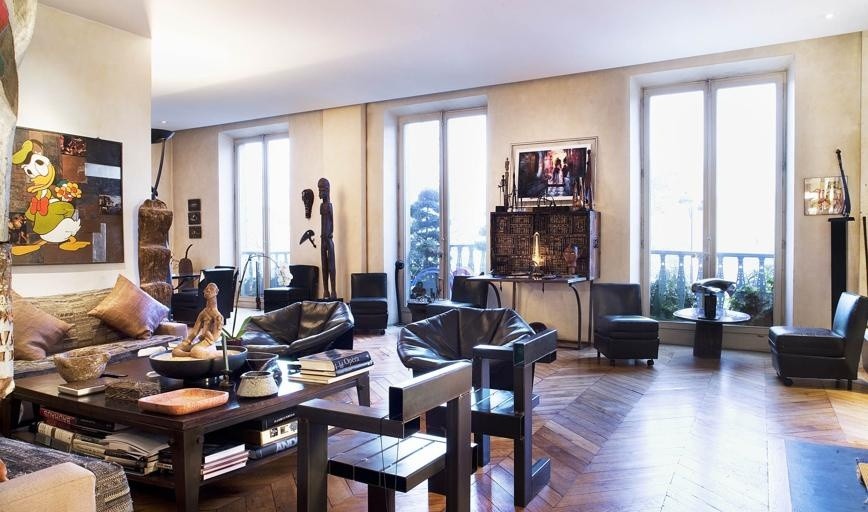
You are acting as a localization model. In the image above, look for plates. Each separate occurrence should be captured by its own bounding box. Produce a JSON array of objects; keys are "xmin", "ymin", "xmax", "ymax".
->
[{"xmin": 138, "ymin": 387, "xmax": 229, "ymax": 417}]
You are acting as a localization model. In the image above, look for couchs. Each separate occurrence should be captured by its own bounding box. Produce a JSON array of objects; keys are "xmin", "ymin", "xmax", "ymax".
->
[{"xmin": 1, "ymin": 285, "xmax": 184, "ymax": 441}]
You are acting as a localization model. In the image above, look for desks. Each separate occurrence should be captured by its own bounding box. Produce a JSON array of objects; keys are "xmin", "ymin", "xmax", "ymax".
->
[
  {"xmin": 672, "ymin": 307, "xmax": 752, "ymax": 360},
  {"xmin": 463, "ymin": 268, "xmax": 594, "ymax": 351}
]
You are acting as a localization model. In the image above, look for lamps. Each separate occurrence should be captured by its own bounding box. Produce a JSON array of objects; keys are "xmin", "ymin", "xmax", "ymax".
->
[
  {"xmin": 531, "ymin": 230, "xmax": 541, "ymax": 266},
  {"xmin": 150, "ymin": 127, "xmax": 176, "ymax": 202}
]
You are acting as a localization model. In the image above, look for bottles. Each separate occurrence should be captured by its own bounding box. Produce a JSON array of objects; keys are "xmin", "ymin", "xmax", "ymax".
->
[
  {"xmin": 237, "ymin": 370, "xmax": 279, "ymax": 399},
  {"xmin": 242, "ymin": 352, "xmax": 283, "ymax": 379}
]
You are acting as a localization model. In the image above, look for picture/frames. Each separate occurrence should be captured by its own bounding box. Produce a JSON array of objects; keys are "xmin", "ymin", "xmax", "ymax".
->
[
  {"xmin": 188, "ymin": 226, "xmax": 201, "ymax": 239},
  {"xmin": 507, "ymin": 135, "xmax": 599, "ymax": 209},
  {"xmin": 802, "ymin": 174, "xmax": 849, "ymax": 217},
  {"xmin": 188, "ymin": 199, "xmax": 201, "ymax": 211},
  {"xmin": 188, "ymin": 212, "xmax": 201, "ymax": 225}
]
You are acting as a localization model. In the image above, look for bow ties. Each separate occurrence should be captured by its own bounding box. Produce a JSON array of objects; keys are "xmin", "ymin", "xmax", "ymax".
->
[{"xmin": 29, "ymin": 195, "xmax": 50, "ymax": 218}]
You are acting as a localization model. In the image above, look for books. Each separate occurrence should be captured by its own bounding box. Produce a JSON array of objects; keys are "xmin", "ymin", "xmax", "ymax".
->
[
  {"xmin": 288, "ymin": 348, "xmax": 375, "ymax": 384},
  {"xmin": 31, "ymin": 404, "xmax": 337, "ymax": 482}
]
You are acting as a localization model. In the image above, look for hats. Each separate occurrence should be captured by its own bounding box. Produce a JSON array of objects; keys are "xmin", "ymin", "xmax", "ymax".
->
[{"xmin": 12, "ymin": 138, "xmax": 35, "ymax": 165}]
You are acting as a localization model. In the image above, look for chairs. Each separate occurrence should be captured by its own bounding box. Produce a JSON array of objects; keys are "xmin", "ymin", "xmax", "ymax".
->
[
  {"xmin": 424, "ymin": 273, "xmax": 490, "ymax": 319},
  {"xmin": 590, "ymin": 282, "xmax": 660, "ymax": 368},
  {"xmin": 295, "ymin": 355, "xmax": 475, "ymax": 511},
  {"xmin": 767, "ymin": 290, "xmax": 868, "ymax": 390},
  {"xmin": 232, "ymin": 298, "xmax": 356, "ymax": 360},
  {"xmin": 169, "ymin": 261, "xmax": 388, "ymax": 336},
  {"xmin": 420, "ymin": 325, "xmax": 563, "ymax": 509},
  {"xmin": 394, "ymin": 302, "xmax": 540, "ymax": 444}
]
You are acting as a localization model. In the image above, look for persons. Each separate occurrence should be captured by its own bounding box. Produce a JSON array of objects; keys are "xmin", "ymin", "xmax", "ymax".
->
[
  {"xmin": 540, "ymin": 155, "xmax": 575, "ymax": 195},
  {"xmin": 317, "ymin": 178, "xmax": 337, "ymax": 300},
  {"xmin": 172, "ymin": 282, "xmax": 225, "ymax": 360},
  {"xmin": 561, "ymin": 242, "xmax": 584, "ymax": 274}
]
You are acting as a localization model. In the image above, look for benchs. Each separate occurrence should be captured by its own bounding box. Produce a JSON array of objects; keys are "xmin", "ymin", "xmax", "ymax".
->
[{"xmin": 0, "ymin": 433, "xmax": 135, "ymax": 511}]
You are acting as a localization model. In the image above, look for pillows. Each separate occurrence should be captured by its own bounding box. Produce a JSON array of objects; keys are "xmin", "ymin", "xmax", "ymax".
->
[
  {"xmin": 85, "ymin": 272, "xmax": 171, "ymax": 342},
  {"xmin": 7, "ymin": 288, "xmax": 78, "ymax": 362}
]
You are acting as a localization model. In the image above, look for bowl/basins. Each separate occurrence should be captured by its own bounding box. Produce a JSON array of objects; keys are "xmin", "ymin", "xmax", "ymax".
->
[
  {"xmin": 149, "ymin": 345, "xmax": 248, "ymax": 388},
  {"xmin": 53, "ymin": 352, "xmax": 107, "ymax": 382}
]
